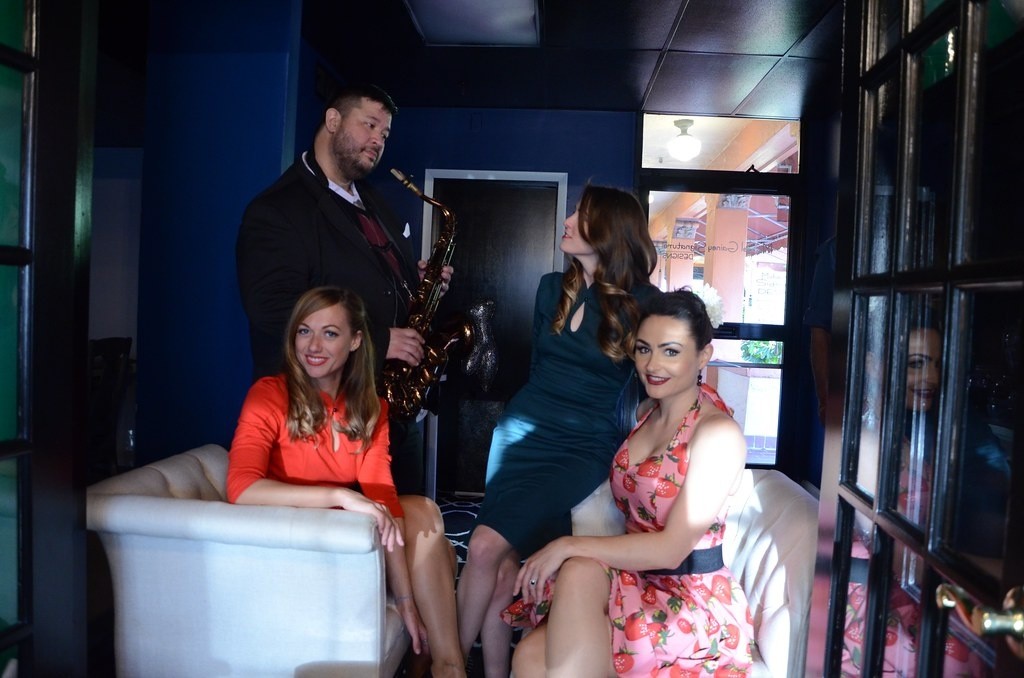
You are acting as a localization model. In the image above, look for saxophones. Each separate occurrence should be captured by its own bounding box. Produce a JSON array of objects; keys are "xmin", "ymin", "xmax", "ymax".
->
[{"xmin": 379, "ymin": 160, "xmax": 477, "ymax": 425}]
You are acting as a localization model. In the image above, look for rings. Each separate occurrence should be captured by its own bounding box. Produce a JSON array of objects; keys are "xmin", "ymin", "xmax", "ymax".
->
[
  {"xmin": 421, "ymin": 639, "xmax": 428, "ymax": 643},
  {"xmin": 528, "ymin": 579, "xmax": 536, "ymax": 586}
]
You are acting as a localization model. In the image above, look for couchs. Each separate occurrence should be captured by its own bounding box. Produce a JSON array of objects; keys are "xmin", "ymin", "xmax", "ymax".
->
[
  {"xmin": 571, "ymin": 469, "xmax": 817, "ymax": 678},
  {"xmin": 86, "ymin": 443, "xmax": 411, "ymax": 678}
]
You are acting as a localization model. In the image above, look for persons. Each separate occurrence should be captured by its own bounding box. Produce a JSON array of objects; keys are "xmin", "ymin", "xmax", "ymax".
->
[
  {"xmin": 842, "ymin": 321, "xmax": 986, "ymax": 678},
  {"xmin": 455, "ymin": 184, "xmax": 663, "ymax": 678},
  {"xmin": 235, "ymin": 78, "xmax": 454, "ymax": 496},
  {"xmin": 225, "ymin": 285, "xmax": 469, "ymax": 678},
  {"xmin": 500, "ymin": 287, "xmax": 756, "ymax": 678}
]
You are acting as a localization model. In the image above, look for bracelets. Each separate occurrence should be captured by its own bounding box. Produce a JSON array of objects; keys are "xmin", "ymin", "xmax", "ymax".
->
[{"xmin": 394, "ymin": 595, "xmax": 413, "ymax": 605}]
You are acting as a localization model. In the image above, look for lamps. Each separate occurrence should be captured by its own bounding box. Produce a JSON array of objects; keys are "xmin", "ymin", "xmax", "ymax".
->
[{"xmin": 667, "ymin": 119, "xmax": 701, "ymax": 161}]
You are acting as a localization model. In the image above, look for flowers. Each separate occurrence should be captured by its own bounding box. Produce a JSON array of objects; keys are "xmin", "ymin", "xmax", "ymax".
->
[{"xmin": 688, "ymin": 283, "xmax": 724, "ymax": 329}]
